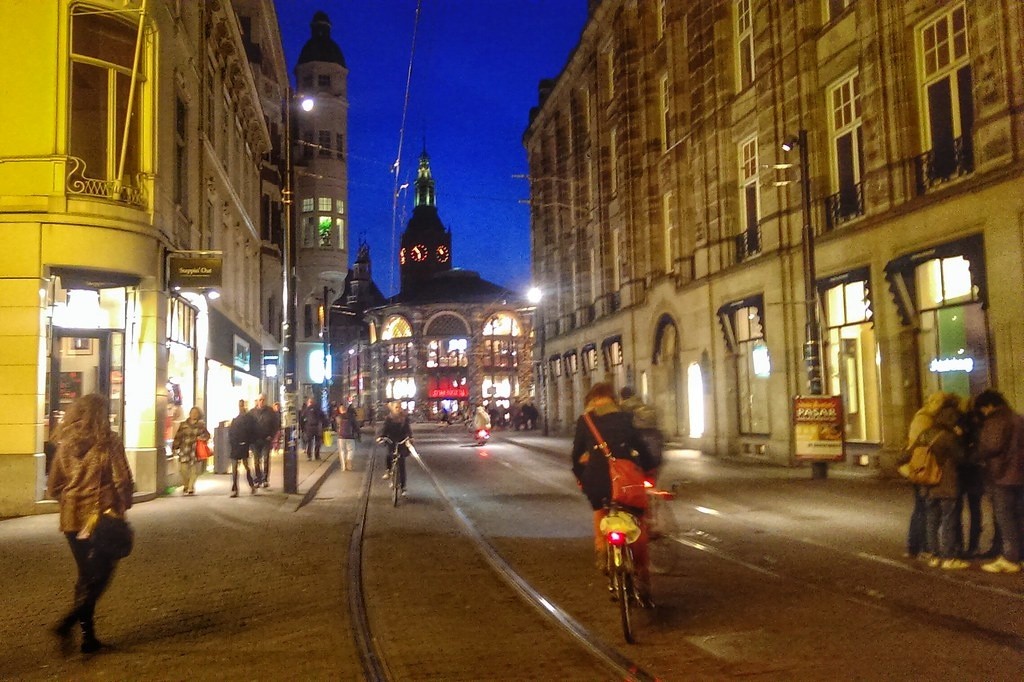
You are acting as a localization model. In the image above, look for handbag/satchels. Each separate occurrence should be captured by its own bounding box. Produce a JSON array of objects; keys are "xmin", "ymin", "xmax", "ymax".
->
[
  {"xmin": 195, "ymin": 440, "xmax": 212, "ymax": 462},
  {"xmin": 610, "ymin": 459, "xmax": 648, "ymax": 509},
  {"xmin": 89, "ymin": 515, "xmax": 132, "ymax": 560}
]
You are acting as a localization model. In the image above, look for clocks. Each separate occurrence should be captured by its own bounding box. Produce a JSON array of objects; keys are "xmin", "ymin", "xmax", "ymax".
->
[{"xmin": 399, "ymin": 244, "xmax": 449, "ymax": 266}]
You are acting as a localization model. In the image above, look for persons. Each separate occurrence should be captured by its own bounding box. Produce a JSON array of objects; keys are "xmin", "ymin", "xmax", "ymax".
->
[
  {"xmin": 46, "ymin": 393, "xmax": 134, "ymax": 657},
  {"xmin": 572, "ymin": 384, "xmax": 661, "ymax": 608},
  {"xmin": 229, "ymin": 394, "xmax": 282, "ymax": 498},
  {"xmin": 376, "ymin": 401, "xmax": 415, "ymax": 496},
  {"xmin": 298, "ymin": 399, "xmax": 327, "ymax": 461},
  {"xmin": 165, "ymin": 377, "xmax": 183, "ymax": 454},
  {"xmin": 908, "ymin": 390, "xmax": 1024, "ymax": 572},
  {"xmin": 440, "ymin": 403, "xmax": 539, "ymax": 440},
  {"xmin": 327, "ymin": 400, "xmax": 374, "ymax": 471},
  {"xmin": 172, "ymin": 407, "xmax": 210, "ymax": 497}
]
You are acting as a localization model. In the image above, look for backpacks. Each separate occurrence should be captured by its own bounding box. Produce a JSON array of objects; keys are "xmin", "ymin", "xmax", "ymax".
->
[{"xmin": 910, "ymin": 430, "xmax": 945, "ymax": 485}]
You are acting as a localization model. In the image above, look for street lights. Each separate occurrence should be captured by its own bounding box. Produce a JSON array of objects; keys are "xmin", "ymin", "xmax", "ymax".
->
[
  {"xmin": 782, "ymin": 129, "xmax": 828, "ymax": 479},
  {"xmin": 282, "ymin": 83, "xmax": 314, "ymax": 494},
  {"xmin": 527, "ymin": 289, "xmax": 548, "ymax": 436}
]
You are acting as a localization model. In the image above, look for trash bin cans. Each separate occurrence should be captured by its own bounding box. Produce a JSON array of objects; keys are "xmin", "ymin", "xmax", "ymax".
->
[{"xmin": 213, "ymin": 421, "xmax": 231, "ymax": 475}]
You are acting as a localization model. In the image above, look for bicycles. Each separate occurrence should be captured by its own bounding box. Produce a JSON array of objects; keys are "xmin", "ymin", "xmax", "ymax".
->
[
  {"xmin": 377, "ymin": 435, "xmax": 413, "ymax": 508},
  {"xmin": 599, "ymin": 477, "xmax": 679, "ymax": 643}
]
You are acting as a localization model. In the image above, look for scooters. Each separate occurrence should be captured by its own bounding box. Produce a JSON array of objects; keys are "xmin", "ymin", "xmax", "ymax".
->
[{"xmin": 474, "ymin": 428, "xmax": 490, "ymax": 446}]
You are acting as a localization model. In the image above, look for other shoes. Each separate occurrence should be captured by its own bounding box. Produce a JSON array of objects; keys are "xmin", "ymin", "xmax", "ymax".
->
[
  {"xmin": 254, "ymin": 482, "xmax": 259, "ymax": 487},
  {"xmin": 262, "ymin": 483, "xmax": 268, "ymax": 488},
  {"xmin": 983, "ymin": 557, "xmax": 1019, "ymax": 573},
  {"xmin": 382, "ymin": 474, "xmax": 390, "ymax": 479},
  {"xmin": 230, "ymin": 491, "xmax": 238, "ymax": 497},
  {"xmin": 81, "ymin": 640, "xmax": 100, "ymax": 658},
  {"xmin": 941, "ymin": 558, "xmax": 970, "ymax": 569},
  {"xmin": 927, "ymin": 557, "xmax": 940, "ymax": 566},
  {"xmin": 403, "ymin": 491, "xmax": 409, "ymax": 497},
  {"xmin": 50, "ymin": 620, "xmax": 73, "ymax": 657}
]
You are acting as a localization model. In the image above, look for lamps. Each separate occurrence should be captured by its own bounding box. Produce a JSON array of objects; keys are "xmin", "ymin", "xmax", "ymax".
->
[{"xmin": 200, "ymin": 289, "xmax": 220, "ymax": 300}]
